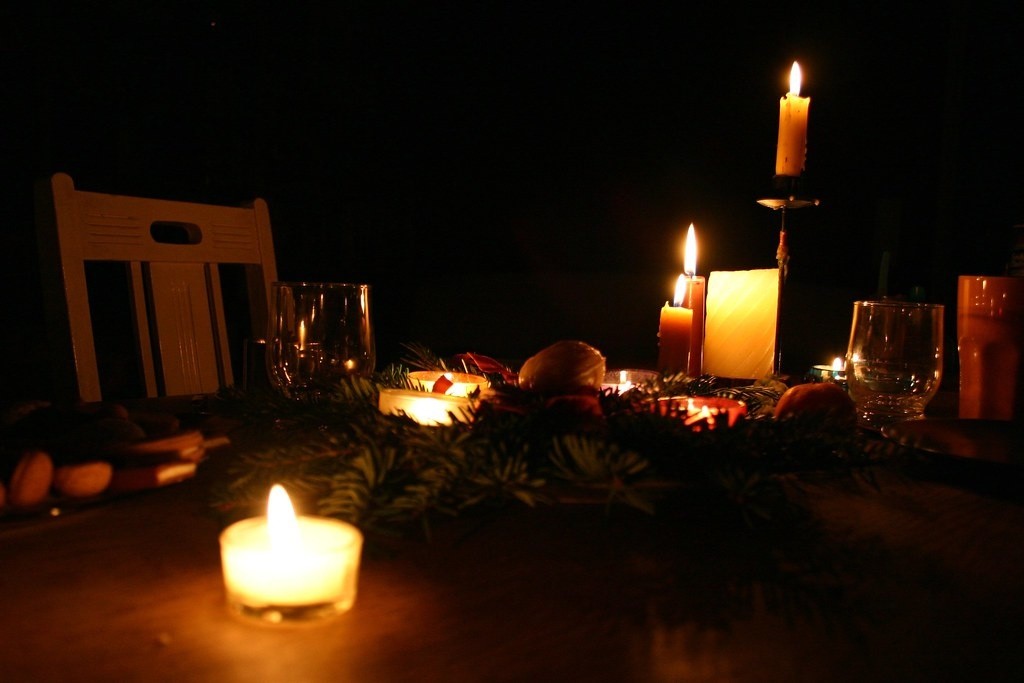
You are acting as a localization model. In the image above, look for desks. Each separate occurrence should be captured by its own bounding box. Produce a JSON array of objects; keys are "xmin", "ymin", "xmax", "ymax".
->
[{"xmin": 0, "ymin": 391, "xmax": 1024, "ymax": 683}]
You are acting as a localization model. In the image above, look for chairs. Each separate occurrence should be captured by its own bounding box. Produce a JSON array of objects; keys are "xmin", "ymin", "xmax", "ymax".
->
[{"xmin": 34, "ymin": 173, "xmax": 278, "ymax": 407}]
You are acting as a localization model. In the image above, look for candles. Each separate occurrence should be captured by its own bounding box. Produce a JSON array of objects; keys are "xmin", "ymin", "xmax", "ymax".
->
[
  {"xmin": 774, "ymin": 59, "xmax": 812, "ymax": 174},
  {"xmin": 658, "ymin": 274, "xmax": 695, "ymax": 383},
  {"xmin": 811, "ymin": 356, "xmax": 845, "ymax": 378},
  {"xmin": 221, "ymin": 483, "xmax": 365, "ymax": 627},
  {"xmin": 682, "ymin": 220, "xmax": 707, "ymax": 378},
  {"xmin": 380, "ymin": 387, "xmax": 473, "ymax": 429},
  {"xmin": 406, "ymin": 372, "xmax": 489, "ymax": 396}
]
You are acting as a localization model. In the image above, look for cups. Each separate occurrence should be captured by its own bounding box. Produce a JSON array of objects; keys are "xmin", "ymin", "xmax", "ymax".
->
[
  {"xmin": 955, "ymin": 275, "xmax": 1024, "ymax": 420},
  {"xmin": 810, "ymin": 364, "xmax": 854, "ymax": 415},
  {"xmin": 267, "ymin": 282, "xmax": 374, "ymax": 425},
  {"xmin": 848, "ymin": 301, "xmax": 945, "ymax": 428},
  {"xmin": 378, "ymin": 389, "xmax": 480, "ymax": 427},
  {"xmin": 657, "ymin": 396, "xmax": 747, "ymax": 432},
  {"xmin": 602, "ymin": 372, "xmax": 659, "ymax": 394},
  {"xmin": 407, "ymin": 363, "xmax": 491, "ymax": 397},
  {"xmin": 222, "ymin": 514, "xmax": 363, "ymax": 630}
]
